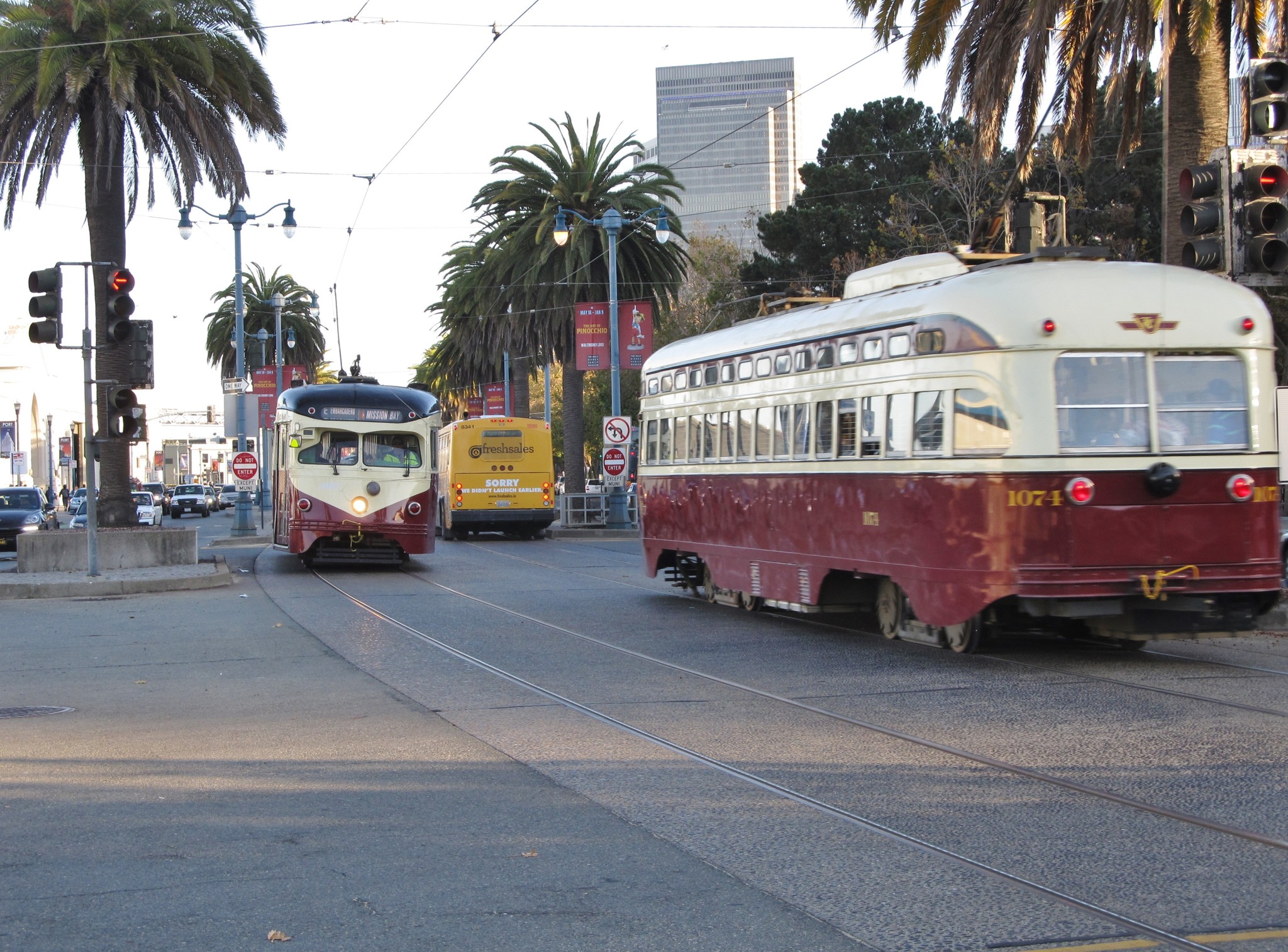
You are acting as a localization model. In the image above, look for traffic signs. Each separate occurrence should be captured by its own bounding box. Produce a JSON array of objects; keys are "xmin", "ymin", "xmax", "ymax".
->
[{"xmin": 222, "ymin": 377, "xmax": 250, "ymax": 394}]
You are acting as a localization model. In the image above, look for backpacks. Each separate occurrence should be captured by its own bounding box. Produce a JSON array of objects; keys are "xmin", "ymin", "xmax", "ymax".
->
[{"xmin": 63, "ymin": 489, "xmax": 68, "ymax": 498}]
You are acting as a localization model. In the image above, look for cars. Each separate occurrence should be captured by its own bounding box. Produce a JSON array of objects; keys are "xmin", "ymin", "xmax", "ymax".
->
[
  {"xmin": 554, "ymin": 475, "xmax": 637, "ymax": 508},
  {"xmin": 68, "ymin": 498, "xmax": 98, "ymax": 529},
  {"xmin": 131, "ymin": 491, "xmax": 162, "ymax": 526}
]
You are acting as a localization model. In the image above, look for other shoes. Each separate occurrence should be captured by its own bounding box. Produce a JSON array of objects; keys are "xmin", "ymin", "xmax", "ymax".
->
[{"xmin": 65, "ymin": 509, "xmax": 67, "ymax": 511}]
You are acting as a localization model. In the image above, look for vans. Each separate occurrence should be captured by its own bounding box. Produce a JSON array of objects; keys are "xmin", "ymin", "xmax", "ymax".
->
[{"xmin": 130, "ymin": 477, "xmax": 142, "ymax": 503}]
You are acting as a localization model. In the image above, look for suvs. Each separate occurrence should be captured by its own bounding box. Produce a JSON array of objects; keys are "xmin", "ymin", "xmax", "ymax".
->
[
  {"xmin": 0, "ymin": 485, "xmax": 59, "ymax": 551},
  {"xmin": 69, "ymin": 487, "xmax": 100, "ymax": 510},
  {"xmin": 141, "ymin": 481, "xmax": 255, "ymax": 519}
]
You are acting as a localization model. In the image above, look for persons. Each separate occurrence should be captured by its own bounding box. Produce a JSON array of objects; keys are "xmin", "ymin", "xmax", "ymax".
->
[
  {"xmin": 384, "ymin": 437, "xmax": 418, "ymax": 466},
  {"xmin": 10, "ymin": 485, "xmax": 86, "ymax": 511}
]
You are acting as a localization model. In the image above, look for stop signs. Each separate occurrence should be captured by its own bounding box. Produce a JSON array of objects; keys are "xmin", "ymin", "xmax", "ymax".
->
[
  {"xmin": 603, "ymin": 448, "xmax": 626, "ymax": 477},
  {"xmin": 231, "ymin": 452, "xmax": 259, "ymax": 480}
]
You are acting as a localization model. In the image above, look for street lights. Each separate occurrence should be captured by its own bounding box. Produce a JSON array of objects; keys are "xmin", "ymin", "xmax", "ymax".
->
[
  {"xmin": 70, "ymin": 422, "xmax": 76, "ymax": 489},
  {"xmin": 552, "ymin": 204, "xmax": 671, "ymax": 531},
  {"xmin": 14, "ymin": 400, "xmax": 21, "ymax": 487},
  {"xmin": 176, "ymin": 440, "xmax": 179, "ymax": 484},
  {"xmin": 46, "ymin": 413, "xmax": 53, "ymax": 505},
  {"xmin": 186, "ymin": 442, "xmax": 191, "ymax": 489},
  {"xmin": 242, "ymin": 288, "xmax": 320, "ymax": 398},
  {"xmin": 178, "ymin": 198, "xmax": 298, "ymax": 538},
  {"xmin": 218, "ymin": 451, "xmax": 221, "ymax": 484},
  {"xmin": 199, "ymin": 447, "xmax": 202, "ymax": 484},
  {"xmin": 162, "ymin": 440, "xmax": 166, "ymax": 484},
  {"xmin": 230, "ymin": 326, "xmax": 296, "ymax": 512},
  {"xmin": 147, "ymin": 437, "xmax": 150, "ymax": 483}
]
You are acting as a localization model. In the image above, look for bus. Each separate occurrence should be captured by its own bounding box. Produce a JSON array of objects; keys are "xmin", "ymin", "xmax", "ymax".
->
[
  {"xmin": 635, "ymin": 2, "xmax": 1279, "ymax": 654},
  {"xmin": 273, "ymin": 283, "xmax": 443, "ymax": 570},
  {"xmin": 435, "ymin": 412, "xmax": 555, "ymax": 541}
]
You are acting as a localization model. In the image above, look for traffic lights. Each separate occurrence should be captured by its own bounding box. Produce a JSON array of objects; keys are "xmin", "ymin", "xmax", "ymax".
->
[
  {"xmin": 598, "ymin": 454, "xmax": 602, "ymax": 459},
  {"xmin": 1250, "ymin": 59, "xmax": 1288, "ymax": 136},
  {"xmin": 106, "ymin": 384, "xmax": 138, "ymax": 439},
  {"xmin": 106, "ymin": 265, "xmax": 136, "ymax": 346},
  {"xmin": 28, "ymin": 266, "xmax": 63, "ymax": 344},
  {"xmin": 1243, "ymin": 162, "xmax": 1288, "ymax": 274},
  {"xmin": 1178, "ymin": 159, "xmax": 1226, "ymax": 274}
]
